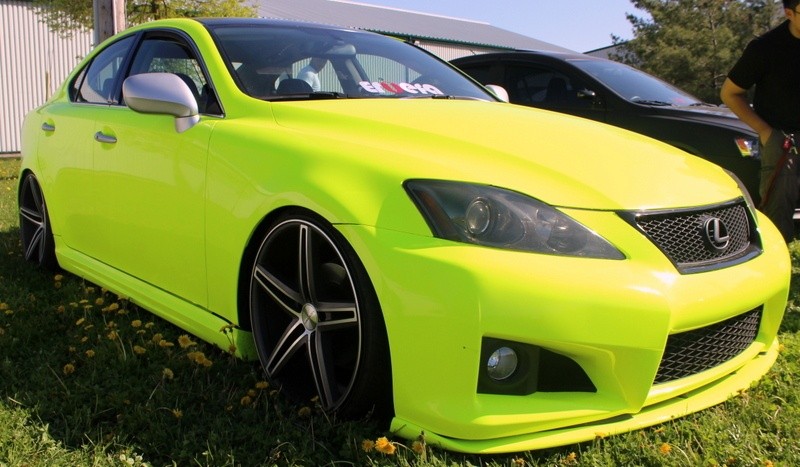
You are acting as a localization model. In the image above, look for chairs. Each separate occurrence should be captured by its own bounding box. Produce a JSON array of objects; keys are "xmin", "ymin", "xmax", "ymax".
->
[{"xmin": 203, "ymin": 78, "xmax": 312, "ymax": 97}]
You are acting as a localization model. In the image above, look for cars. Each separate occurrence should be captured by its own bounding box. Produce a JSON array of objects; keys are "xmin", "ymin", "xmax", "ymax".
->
[
  {"xmin": 14, "ymin": 16, "xmax": 793, "ymax": 454},
  {"xmin": 392, "ymin": 47, "xmax": 764, "ymax": 212}
]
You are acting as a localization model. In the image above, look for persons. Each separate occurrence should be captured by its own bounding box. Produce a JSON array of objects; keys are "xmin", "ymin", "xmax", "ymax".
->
[
  {"xmin": 297, "ymin": 57, "xmax": 327, "ymax": 91},
  {"xmin": 720, "ymin": 0, "xmax": 800, "ymax": 241}
]
